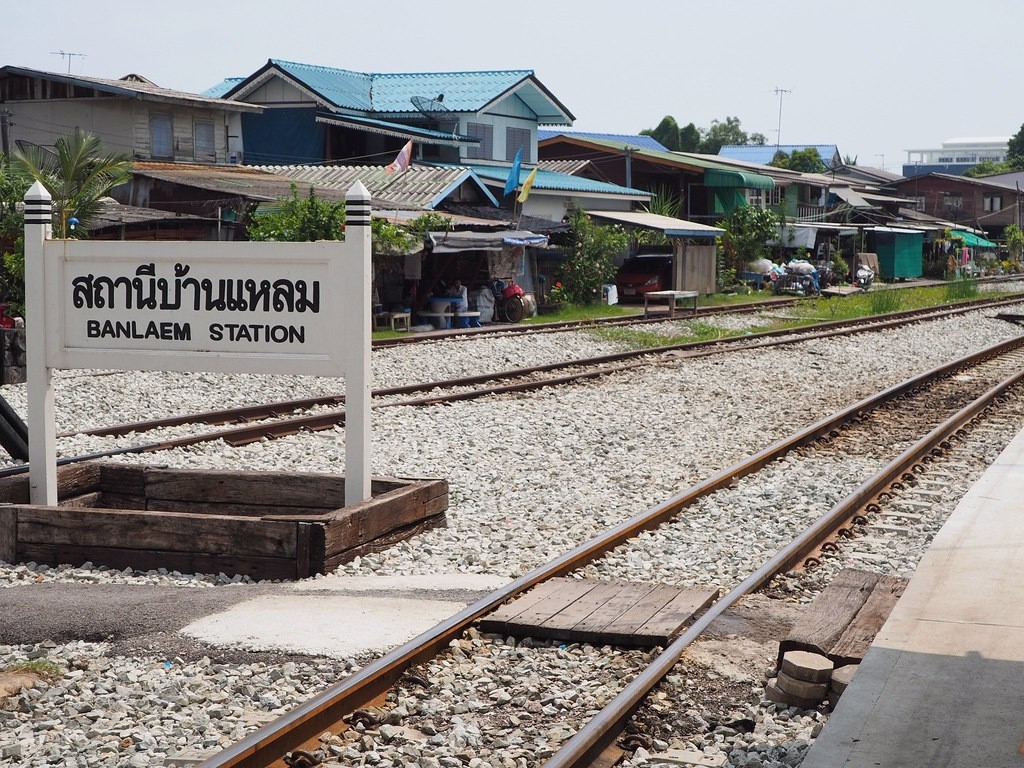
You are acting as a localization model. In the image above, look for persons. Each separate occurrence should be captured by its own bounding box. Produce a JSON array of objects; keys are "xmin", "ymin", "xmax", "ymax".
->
[{"xmin": 444, "ymin": 277, "xmax": 468, "ymax": 320}]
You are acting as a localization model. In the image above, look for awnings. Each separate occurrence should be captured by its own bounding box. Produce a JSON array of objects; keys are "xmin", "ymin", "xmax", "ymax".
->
[
  {"xmin": 704, "ymin": 170, "xmax": 775, "ymax": 190},
  {"xmin": 409, "ymin": 231, "xmax": 551, "ymax": 253},
  {"xmin": 948, "ymin": 231, "xmax": 997, "ymax": 246}
]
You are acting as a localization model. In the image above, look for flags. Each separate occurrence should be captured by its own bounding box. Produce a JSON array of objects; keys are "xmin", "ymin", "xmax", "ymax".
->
[
  {"xmin": 385, "ymin": 138, "xmax": 412, "ymax": 175},
  {"xmin": 517, "ymin": 166, "xmax": 537, "ymax": 203},
  {"xmin": 503, "ymin": 146, "xmax": 522, "ymax": 196}
]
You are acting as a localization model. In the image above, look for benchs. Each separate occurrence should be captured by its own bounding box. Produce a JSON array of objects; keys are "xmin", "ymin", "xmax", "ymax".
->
[
  {"xmin": 454, "ymin": 310, "xmax": 482, "ymax": 329},
  {"xmin": 373, "ymin": 310, "xmax": 411, "ymax": 332}
]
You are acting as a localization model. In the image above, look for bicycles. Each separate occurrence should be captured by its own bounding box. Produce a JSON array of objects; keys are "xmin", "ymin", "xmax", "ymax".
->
[{"xmin": 496, "ymin": 293, "xmax": 525, "ymax": 323}]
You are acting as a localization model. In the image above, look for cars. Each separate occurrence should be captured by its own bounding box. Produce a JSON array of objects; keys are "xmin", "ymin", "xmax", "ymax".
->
[{"xmin": 613, "ymin": 257, "xmax": 673, "ymax": 305}]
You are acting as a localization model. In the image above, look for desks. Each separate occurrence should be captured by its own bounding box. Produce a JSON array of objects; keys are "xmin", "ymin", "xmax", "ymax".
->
[
  {"xmin": 416, "ymin": 295, "xmax": 463, "ymax": 329},
  {"xmin": 643, "ymin": 290, "xmax": 699, "ymax": 319},
  {"xmin": 744, "ymin": 271, "xmax": 770, "ymax": 291},
  {"xmin": 774, "ymin": 274, "xmax": 804, "ymax": 292}
]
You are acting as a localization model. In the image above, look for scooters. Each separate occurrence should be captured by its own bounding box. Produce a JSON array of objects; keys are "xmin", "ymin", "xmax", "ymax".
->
[
  {"xmin": 801, "ymin": 269, "xmax": 822, "ymax": 297},
  {"xmin": 856, "ymin": 259, "xmax": 876, "ymax": 291}
]
